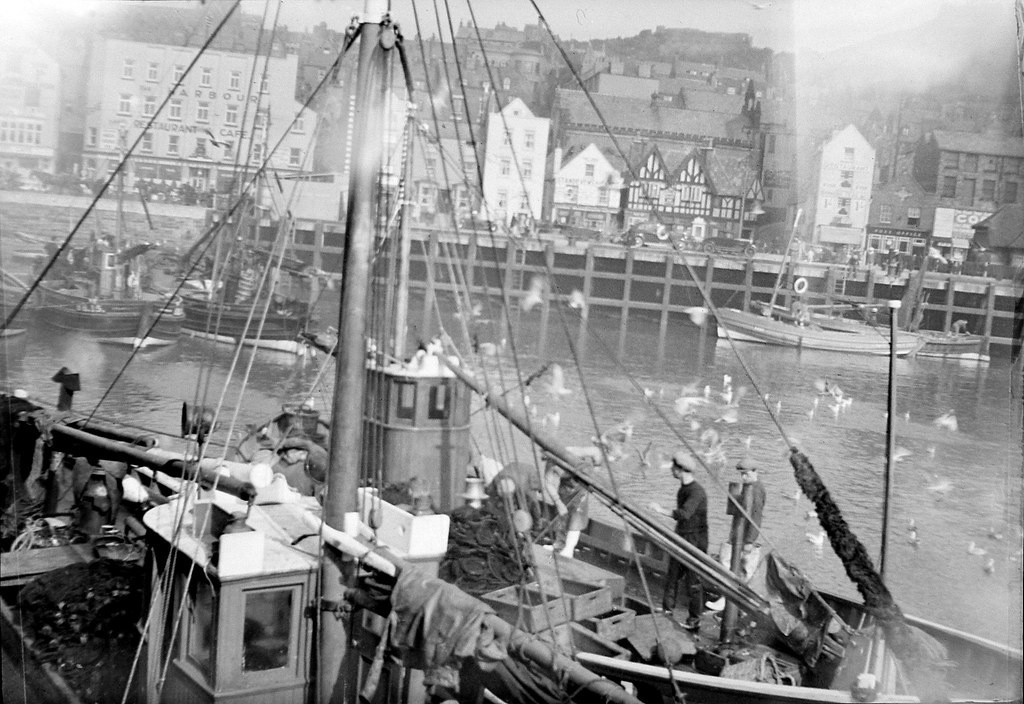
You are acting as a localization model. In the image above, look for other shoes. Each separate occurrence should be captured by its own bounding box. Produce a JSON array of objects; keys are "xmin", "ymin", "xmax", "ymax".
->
[
  {"xmin": 543, "ymin": 540, "xmax": 565, "ymax": 551},
  {"xmin": 705, "ymin": 597, "xmax": 726, "ymax": 611}
]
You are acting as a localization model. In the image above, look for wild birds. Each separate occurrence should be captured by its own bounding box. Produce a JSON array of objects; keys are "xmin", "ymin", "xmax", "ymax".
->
[{"xmin": 407, "ymin": 278, "xmax": 1023, "ymax": 577}]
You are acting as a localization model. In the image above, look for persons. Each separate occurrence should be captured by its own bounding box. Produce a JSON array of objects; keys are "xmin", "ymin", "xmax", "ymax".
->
[
  {"xmin": 705, "ymin": 458, "xmax": 767, "ymax": 611},
  {"xmin": 45, "ymin": 235, "xmax": 75, "ymax": 278},
  {"xmin": 543, "ymin": 444, "xmax": 605, "ymax": 559},
  {"xmin": 660, "ymin": 452, "xmax": 707, "ymax": 632}
]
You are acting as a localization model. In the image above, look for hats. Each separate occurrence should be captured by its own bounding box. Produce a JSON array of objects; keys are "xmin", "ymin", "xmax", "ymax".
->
[
  {"xmin": 496, "ymin": 478, "xmax": 516, "ymax": 498},
  {"xmin": 672, "ymin": 451, "xmax": 696, "ymax": 473},
  {"xmin": 736, "ymin": 458, "xmax": 759, "ymax": 472}
]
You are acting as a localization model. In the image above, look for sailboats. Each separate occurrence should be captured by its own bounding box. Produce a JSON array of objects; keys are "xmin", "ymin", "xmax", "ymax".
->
[{"xmin": 0, "ymin": 0, "xmax": 1024, "ymax": 704}]
